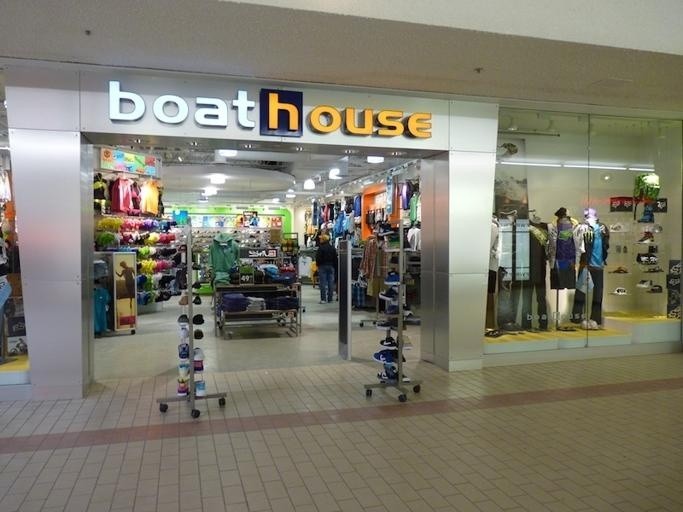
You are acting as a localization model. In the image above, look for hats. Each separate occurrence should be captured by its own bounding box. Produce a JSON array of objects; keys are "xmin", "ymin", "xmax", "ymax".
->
[
  {"xmin": 647, "ymin": 285, "xmax": 662, "ymax": 293},
  {"xmin": 608, "ymin": 288, "xmax": 628, "ymax": 295}
]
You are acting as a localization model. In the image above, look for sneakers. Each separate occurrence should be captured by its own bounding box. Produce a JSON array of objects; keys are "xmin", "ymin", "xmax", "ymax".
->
[
  {"xmin": 403, "ymin": 310, "xmax": 415, "ymax": 321},
  {"xmin": 378, "ymin": 286, "xmax": 397, "ymax": 301},
  {"xmin": 177, "ymin": 377, "xmax": 188, "ymax": 401},
  {"xmin": 177, "ymin": 314, "xmax": 189, "ymax": 339},
  {"xmin": 384, "ymin": 271, "xmax": 400, "ymax": 286},
  {"xmin": 193, "ymin": 314, "xmax": 204, "ymax": 325},
  {"xmin": 372, "ymin": 349, "xmax": 407, "ymax": 363},
  {"xmin": 193, "ymin": 282, "xmax": 202, "ymax": 289},
  {"xmin": 192, "ymin": 296, "xmax": 202, "ymax": 305},
  {"xmin": 378, "ymin": 364, "xmax": 414, "ymax": 385},
  {"xmin": 380, "ymin": 334, "xmax": 414, "ymax": 349},
  {"xmin": 375, "ymin": 319, "xmax": 392, "ymax": 330},
  {"xmin": 382, "ymin": 305, "xmax": 399, "ymax": 318},
  {"xmin": 556, "ymin": 319, "xmax": 599, "ymax": 331},
  {"xmin": 637, "ymin": 212, "xmax": 657, "ymax": 264},
  {"xmin": 179, "ymin": 295, "xmax": 187, "ymax": 306},
  {"xmin": 192, "ymin": 264, "xmax": 201, "ymax": 270},
  {"xmin": 177, "ymin": 342, "xmax": 190, "ymax": 376},
  {"xmin": 485, "ymin": 322, "xmax": 527, "ymax": 338},
  {"xmin": 636, "ymin": 279, "xmax": 653, "ymax": 287},
  {"xmin": 192, "ymin": 347, "xmax": 205, "ymax": 375},
  {"xmin": 195, "ymin": 379, "xmax": 207, "ymax": 399},
  {"xmin": 609, "ymin": 267, "xmax": 628, "ymax": 273},
  {"xmin": 194, "ymin": 329, "xmax": 204, "ymax": 339}
]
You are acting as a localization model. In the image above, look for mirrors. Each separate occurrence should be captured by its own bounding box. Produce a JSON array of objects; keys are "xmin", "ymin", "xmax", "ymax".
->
[{"xmin": 112, "ymin": 252, "xmax": 137, "ymax": 332}]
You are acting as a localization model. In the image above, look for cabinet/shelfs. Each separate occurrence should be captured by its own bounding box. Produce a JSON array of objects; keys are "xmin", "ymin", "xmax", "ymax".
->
[{"xmin": 212, "ymin": 279, "xmax": 303, "ymax": 340}]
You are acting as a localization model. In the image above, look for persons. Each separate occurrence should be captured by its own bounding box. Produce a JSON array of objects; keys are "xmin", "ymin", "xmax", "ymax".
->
[
  {"xmin": 116, "ymin": 260, "xmax": 136, "ymax": 312},
  {"xmin": 315, "ymin": 234, "xmax": 337, "ymax": 304},
  {"xmin": 547, "ymin": 208, "xmax": 584, "ymax": 290},
  {"xmin": 572, "ymin": 209, "xmax": 610, "ymax": 327},
  {"xmin": 518, "ymin": 211, "xmax": 552, "ymax": 332},
  {"xmin": 485, "ymin": 212, "xmax": 504, "ymax": 339}
]
